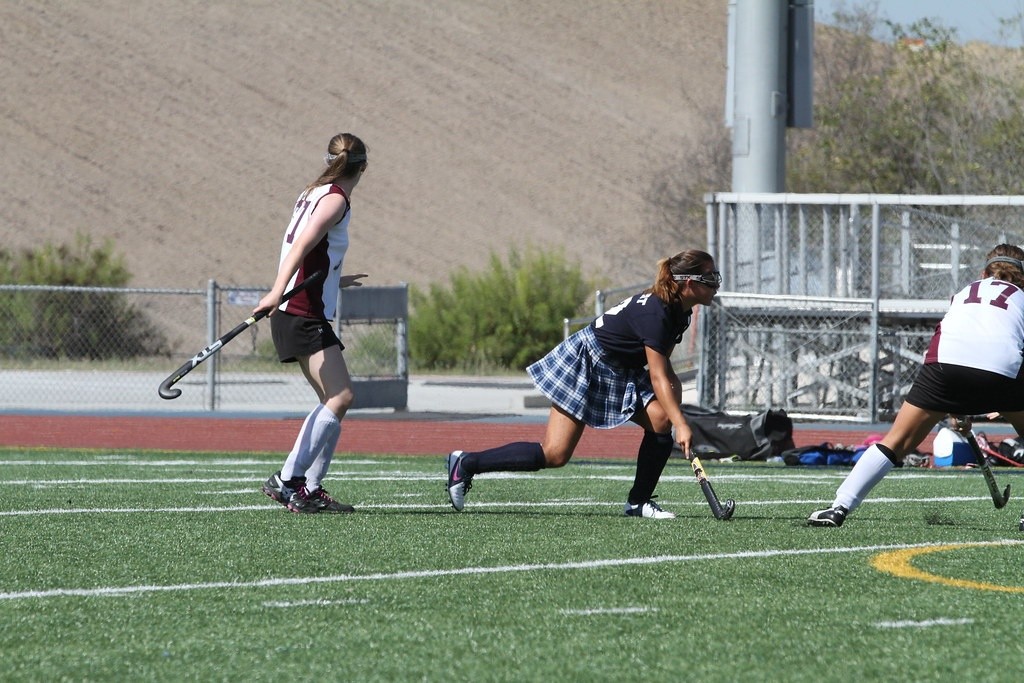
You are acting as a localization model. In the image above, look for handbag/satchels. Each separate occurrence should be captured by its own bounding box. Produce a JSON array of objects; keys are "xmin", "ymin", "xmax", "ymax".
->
[{"xmin": 674, "ymin": 403, "xmax": 795, "ymax": 462}]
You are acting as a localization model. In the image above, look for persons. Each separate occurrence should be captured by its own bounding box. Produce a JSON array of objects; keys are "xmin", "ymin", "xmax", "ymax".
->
[
  {"xmin": 446, "ymin": 249, "xmax": 720, "ymax": 518},
  {"xmin": 253, "ymin": 132, "xmax": 369, "ymax": 514},
  {"xmin": 807, "ymin": 243, "xmax": 1024, "ymax": 528}
]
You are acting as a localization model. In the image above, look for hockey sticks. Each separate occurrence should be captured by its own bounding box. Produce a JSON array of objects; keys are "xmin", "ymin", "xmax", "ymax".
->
[
  {"xmin": 677, "ymin": 439, "xmax": 736, "ymax": 522},
  {"xmin": 953, "ymin": 417, "xmax": 1013, "ymax": 510},
  {"xmin": 157, "ymin": 265, "xmax": 329, "ymax": 400}
]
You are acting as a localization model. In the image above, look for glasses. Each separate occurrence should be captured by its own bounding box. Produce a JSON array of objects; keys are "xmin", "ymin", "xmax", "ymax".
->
[{"xmin": 672, "ymin": 270, "xmax": 725, "ymax": 290}]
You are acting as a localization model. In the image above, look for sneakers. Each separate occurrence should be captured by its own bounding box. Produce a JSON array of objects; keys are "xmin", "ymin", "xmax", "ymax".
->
[
  {"xmin": 262, "ymin": 471, "xmax": 354, "ymax": 514},
  {"xmin": 447, "ymin": 451, "xmax": 470, "ymax": 510},
  {"xmin": 622, "ymin": 499, "xmax": 675, "ymax": 520},
  {"xmin": 808, "ymin": 506, "xmax": 848, "ymax": 528}
]
